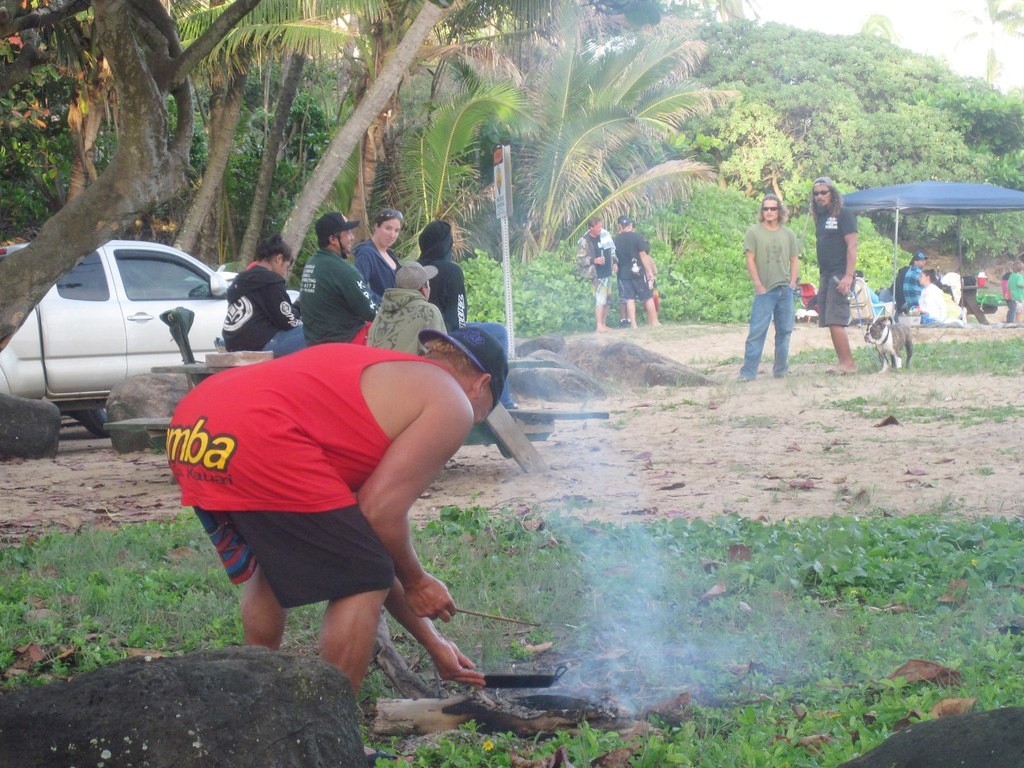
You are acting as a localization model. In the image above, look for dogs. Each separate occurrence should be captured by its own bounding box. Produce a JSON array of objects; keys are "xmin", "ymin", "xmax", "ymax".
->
[{"xmin": 864, "ymin": 320, "xmax": 914, "ymax": 374}]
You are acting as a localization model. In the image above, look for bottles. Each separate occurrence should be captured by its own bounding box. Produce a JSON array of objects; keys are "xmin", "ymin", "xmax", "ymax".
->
[
  {"xmin": 977, "ymin": 272, "xmax": 987, "ymax": 285},
  {"xmin": 648, "ymin": 280, "xmax": 653, "ymax": 290},
  {"xmin": 935, "ymin": 265, "xmax": 941, "ymax": 281},
  {"xmin": 833, "ymin": 276, "xmax": 856, "ymax": 299},
  {"xmin": 597, "ymin": 242, "xmax": 605, "ymax": 258}
]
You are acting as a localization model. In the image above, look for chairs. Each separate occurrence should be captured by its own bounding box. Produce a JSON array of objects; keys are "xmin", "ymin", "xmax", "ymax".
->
[
  {"xmin": 798, "ymin": 283, "xmax": 819, "ymax": 324},
  {"xmin": 850, "ymin": 277, "xmax": 896, "ymax": 334},
  {"xmin": 164, "ymin": 307, "xmax": 207, "ymax": 385}
]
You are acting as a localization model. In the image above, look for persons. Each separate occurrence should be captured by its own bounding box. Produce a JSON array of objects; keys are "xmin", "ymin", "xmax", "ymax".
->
[
  {"xmin": 736, "ymin": 193, "xmax": 798, "ymax": 382},
  {"xmin": 353, "ymin": 208, "xmax": 404, "ymax": 309},
  {"xmin": 612, "ymin": 215, "xmax": 663, "ymax": 328},
  {"xmin": 166, "ymin": 324, "xmax": 509, "ymax": 754},
  {"xmin": 889, "ymin": 250, "xmax": 962, "ymax": 322},
  {"xmin": 222, "ymin": 233, "xmax": 306, "ymax": 359},
  {"xmin": 576, "ymin": 217, "xmax": 619, "ymax": 333},
  {"xmin": 1002, "ymin": 252, "xmax": 1024, "ymax": 323},
  {"xmin": 806, "ymin": 269, "xmax": 885, "ymax": 322},
  {"xmin": 366, "ymin": 262, "xmax": 448, "ymax": 356},
  {"xmin": 877, "ymin": 282, "xmax": 893, "ymax": 303},
  {"xmin": 415, "ymin": 220, "xmax": 468, "ymax": 333},
  {"xmin": 300, "ymin": 212, "xmax": 379, "ymax": 347},
  {"xmin": 812, "ymin": 175, "xmax": 859, "ymax": 374}
]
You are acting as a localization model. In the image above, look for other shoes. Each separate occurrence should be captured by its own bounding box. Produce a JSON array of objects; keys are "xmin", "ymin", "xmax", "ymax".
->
[
  {"xmin": 620, "ymin": 319, "xmax": 630, "ymax": 327},
  {"xmin": 825, "ymin": 365, "xmax": 853, "ymax": 376},
  {"xmin": 737, "ymin": 374, "xmax": 748, "ymax": 384}
]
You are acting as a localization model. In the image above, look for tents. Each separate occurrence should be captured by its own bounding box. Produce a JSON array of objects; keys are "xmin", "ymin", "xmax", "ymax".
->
[{"xmin": 841, "ymin": 181, "xmax": 1024, "ymax": 324}]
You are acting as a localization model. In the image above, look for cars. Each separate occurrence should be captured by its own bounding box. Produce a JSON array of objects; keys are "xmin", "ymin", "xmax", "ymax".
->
[{"xmin": 0, "ymin": 240, "xmax": 301, "ymax": 437}]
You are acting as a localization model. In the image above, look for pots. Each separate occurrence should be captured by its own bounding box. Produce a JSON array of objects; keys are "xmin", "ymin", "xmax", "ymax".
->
[{"xmin": 462, "ymin": 665, "xmax": 568, "ymax": 688}]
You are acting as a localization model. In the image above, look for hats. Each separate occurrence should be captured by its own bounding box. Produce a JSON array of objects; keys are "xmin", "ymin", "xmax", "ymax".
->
[
  {"xmin": 914, "ymin": 251, "xmax": 928, "ymax": 261},
  {"xmin": 814, "ymin": 177, "xmax": 837, "ymax": 191},
  {"xmin": 619, "ymin": 217, "xmax": 631, "ymax": 228},
  {"xmin": 395, "ymin": 260, "xmax": 438, "ymax": 290},
  {"xmin": 316, "ymin": 212, "xmax": 363, "ymax": 242},
  {"xmin": 419, "ymin": 326, "xmax": 515, "ymax": 415}
]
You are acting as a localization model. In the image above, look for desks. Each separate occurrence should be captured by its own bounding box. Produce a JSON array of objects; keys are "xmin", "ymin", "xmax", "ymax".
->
[{"xmin": 959, "ymin": 286, "xmax": 990, "ymax": 324}]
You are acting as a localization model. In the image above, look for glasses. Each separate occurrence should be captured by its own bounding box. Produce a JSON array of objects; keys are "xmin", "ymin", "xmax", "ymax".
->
[
  {"xmin": 763, "ymin": 206, "xmax": 781, "ymax": 212},
  {"xmin": 378, "ymin": 211, "xmax": 402, "ymax": 224},
  {"xmin": 814, "ymin": 191, "xmax": 831, "ymax": 197}
]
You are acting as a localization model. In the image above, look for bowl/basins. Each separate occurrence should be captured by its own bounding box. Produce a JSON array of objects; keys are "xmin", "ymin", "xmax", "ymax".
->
[
  {"xmin": 982, "ymin": 304, "xmax": 999, "ymax": 312},
  {"xmin": 909, "ymin": 310, "xmax": 920, "ymax": 315}
]
